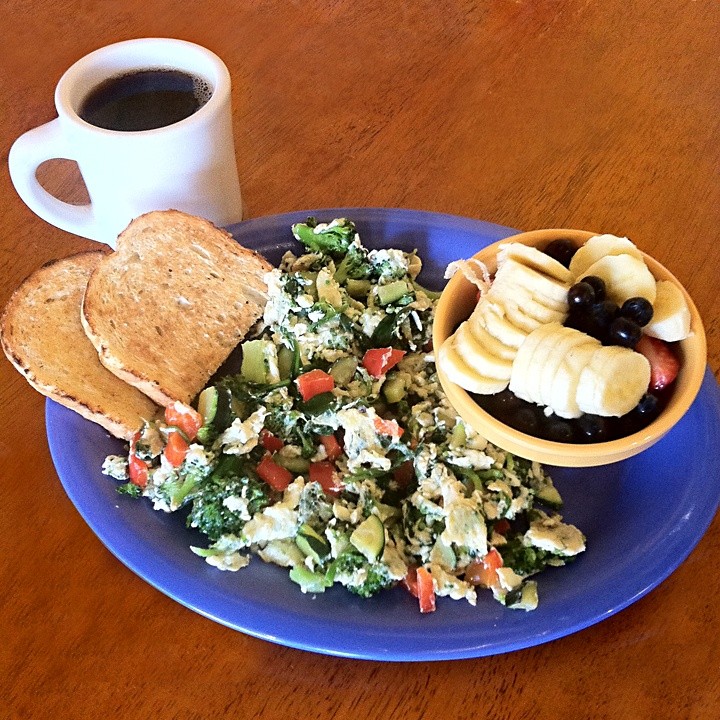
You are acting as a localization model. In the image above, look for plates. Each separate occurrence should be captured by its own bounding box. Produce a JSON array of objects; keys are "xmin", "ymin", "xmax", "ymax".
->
[{"xmin": 43, "ymin": 210, "xmax": 720, "ymax": 660}]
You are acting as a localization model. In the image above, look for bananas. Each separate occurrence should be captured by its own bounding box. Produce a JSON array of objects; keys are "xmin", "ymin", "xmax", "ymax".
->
[{"xmin": 435, "ymin": 231, "xmax": 694, "ymax": 421}]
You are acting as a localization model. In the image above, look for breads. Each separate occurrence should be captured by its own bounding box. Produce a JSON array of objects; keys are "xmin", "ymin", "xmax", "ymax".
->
[
  {"xmin": 81, "ymin": 207, "xmax": 277, "ymax": 409},
  {"xmin": 0, "ymin": 247, "xmax": 162, "ymax": 443}
]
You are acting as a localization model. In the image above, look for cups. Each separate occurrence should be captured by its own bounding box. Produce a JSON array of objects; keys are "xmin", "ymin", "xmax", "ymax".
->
[{"xmin": 7, "ymin": 37, "xmax": 243, "ymax": 251}]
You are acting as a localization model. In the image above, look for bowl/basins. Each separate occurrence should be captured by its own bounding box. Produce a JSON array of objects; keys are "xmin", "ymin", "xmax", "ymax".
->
[{"xmin": 431, "ymin": 229, "xmax": 708, "ymax": 467}]
